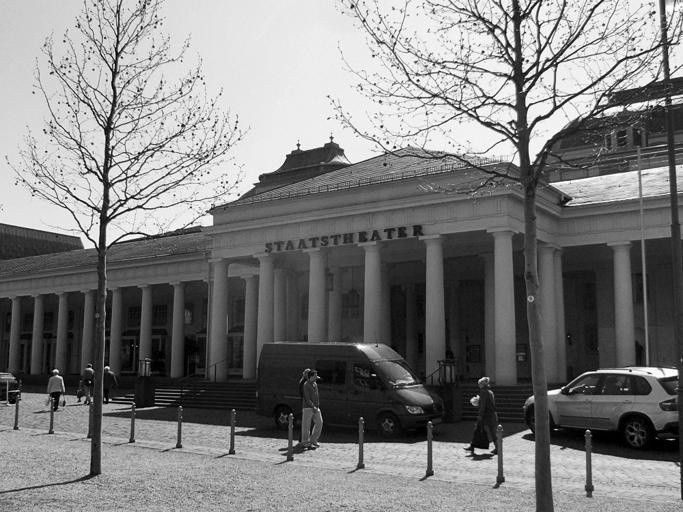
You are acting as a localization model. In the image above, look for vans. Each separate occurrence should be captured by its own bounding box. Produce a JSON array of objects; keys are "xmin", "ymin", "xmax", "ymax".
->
[{"xmin": 255, "ymin": 342, "xmax": 445, "ymax": 440}]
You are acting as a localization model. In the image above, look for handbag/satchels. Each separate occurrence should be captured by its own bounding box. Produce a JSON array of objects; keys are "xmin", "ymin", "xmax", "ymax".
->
[
  {"xmin": 85, "ymin": 378, "xmax": 92, "ymax": 387},
  {"xmin": 471, "ymin": 421, "xmax": 489, "ymax": 449}
]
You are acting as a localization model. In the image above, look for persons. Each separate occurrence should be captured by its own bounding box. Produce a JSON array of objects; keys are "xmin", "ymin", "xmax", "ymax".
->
[
  {"xmin": 47, "ymin": 369, "xmax": 66, "ymax": 411},
  {"xmin": 80, "ymin": 363, "xmax": 94, "ymax": 405},
  {"xmin": 103, "ymin": 365, "xmax": 116, "ymax": 404},
  {"xmin": 301, "ymin": 370, "xmax": 323, "ymax": 450},
  {"xmin": 297, "ymin": 368, "xmax": 314, "ymax": 447},
  {"xmin": 463, "ymin": 377, "xmax": 499, "ymax": 454}
]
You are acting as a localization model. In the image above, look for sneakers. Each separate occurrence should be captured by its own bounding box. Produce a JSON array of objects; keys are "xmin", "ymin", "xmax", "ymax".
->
[{"xmin": 463, "ymin": 446, "xmax": 497, "ymax": 454}]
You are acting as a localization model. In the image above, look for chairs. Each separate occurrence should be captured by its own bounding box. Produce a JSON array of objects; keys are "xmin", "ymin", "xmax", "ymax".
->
[{"xmin": 602, "ymin": 377, "xmax": 619, "ymax": 395}]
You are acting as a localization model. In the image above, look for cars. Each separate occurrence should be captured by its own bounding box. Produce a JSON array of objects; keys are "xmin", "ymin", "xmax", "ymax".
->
[
  {"xmin": 521, "ymin": 366, "xmax": 679, "ymax": 451},
  {"xmin": 0, "ymin": 372, "xmax": 18, "ymax": 400}
]
